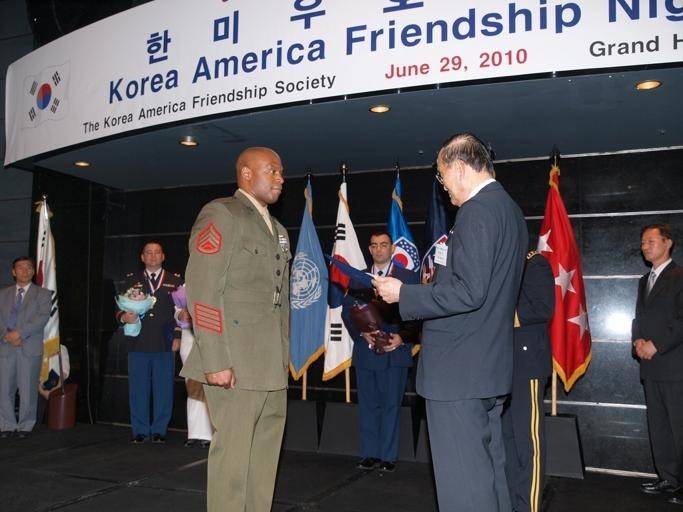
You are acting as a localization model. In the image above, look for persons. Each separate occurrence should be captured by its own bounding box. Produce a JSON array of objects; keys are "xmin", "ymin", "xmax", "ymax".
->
[
  {"xmin": 630, "ymin": 222, "xmax": 683, "ymax": 507},
  {"xmin": 0, "ymin": 256, "xmax": 53, "ymax": 441},
  {"xmin": 177, "ymin": 145, "xmax": 294, "ymax": 512},
  {"xmin": 113, "ymin": 241, "xmax": 184, "ymax": 446},
  {"xmin": 368, "ymin": 130, "xmax": 533, "ymax": 512},
  {"xmin": 501, "ymin": 244, "xmax": 555, "ymax": 512},
  {"xmin": 172, "ymin": 283, "xmax": 214, "ymax": 450},
  {"xmin": 340, "ymin": 229, "xmax": 422, "ymax": 474}
]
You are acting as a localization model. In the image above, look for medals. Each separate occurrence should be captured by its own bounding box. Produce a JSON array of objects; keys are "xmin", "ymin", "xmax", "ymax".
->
[{"xmin": 150, "ymin": 295, "xmax": 157, "ymax": 304}]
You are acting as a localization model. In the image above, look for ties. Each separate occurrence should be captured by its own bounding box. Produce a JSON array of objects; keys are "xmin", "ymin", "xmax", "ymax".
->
[
  {"xmin": 16, "ymin": 288, "xmax": 24, "ymax": 311},
  {"xmin": 378, "ymin": 271, "xmax": 383, "ymax": 275},
  {"xmin": 646, "ymin": 272, "xmax": 656, "ymax": 296},
  {"xmin": 151, "ymin": 273, "xmax": 156, "ymax": 289}
]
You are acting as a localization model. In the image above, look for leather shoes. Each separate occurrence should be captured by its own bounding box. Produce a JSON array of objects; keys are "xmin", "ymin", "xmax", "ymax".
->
[
  {"xmin": 198, "ymin": 440, "xmax": 210, "ymax": 447},
  {"xmin": 640, "ymin": 478, "xmax": 682, "ymax": 495},
  {"xmin": 184, "ymin": 439, "xmax": 198, "ymax": 447},
  {"xmin": 0, "ymin": 431, "xmax": 14, "ymax": 438},
  {"xmin": 356, "ymin": 457, "xmax": 381, "ymax": 471},
  {"xmin": 17, "ymin": 430, "xmax": 31, "ymax": 439},
  {"xmin": 132, "ymin": 434, "xmax": 149, "ymax": 444},
  {"xmin": 664, "ymin": 488, "xmax": 682, "ymax": 505},
  {"xmin": 378, "ymin": 461, "xmax": 398, "ymax": 473},
  {"xmin": 152, "ymin": 434, "xmax": 166, "ymax": 443}
]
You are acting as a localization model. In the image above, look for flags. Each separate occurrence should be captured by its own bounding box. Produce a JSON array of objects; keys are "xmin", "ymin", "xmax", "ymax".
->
[
  {"xmin": 33, "ymin": 195, "xmax": 61, "ymax": 384},
  {"xmin": 536, "ymin": 149, "xmax": 592, "ymax": 393},
  {"xmin": 321, "ymin": 163, "xmax": 375, "ymax": 381},
  {"xmin": 22, "ymin": 59, "xmax": 68, "ymax": 129},
  {"xmin": 423, "ymin": 172, "xmax": 448, "ymax": 257},
  {"xmin": 287, "ymin": 170, "xmax": 328, "ymax": 381},
  {"xmin": 388, "ymin": 166, "xmax": 421, "ymax": 358}
]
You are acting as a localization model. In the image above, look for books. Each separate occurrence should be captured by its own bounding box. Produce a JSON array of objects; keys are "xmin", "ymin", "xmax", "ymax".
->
[{"xmin": 323, "ymin": 253, "xmax": 378, "ymax": 290}]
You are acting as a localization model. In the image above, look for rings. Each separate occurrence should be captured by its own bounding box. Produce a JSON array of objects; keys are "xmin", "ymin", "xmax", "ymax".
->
[{"xmin": 392, "ymin": 347, "xmax": 395, "ymax": 349}]
